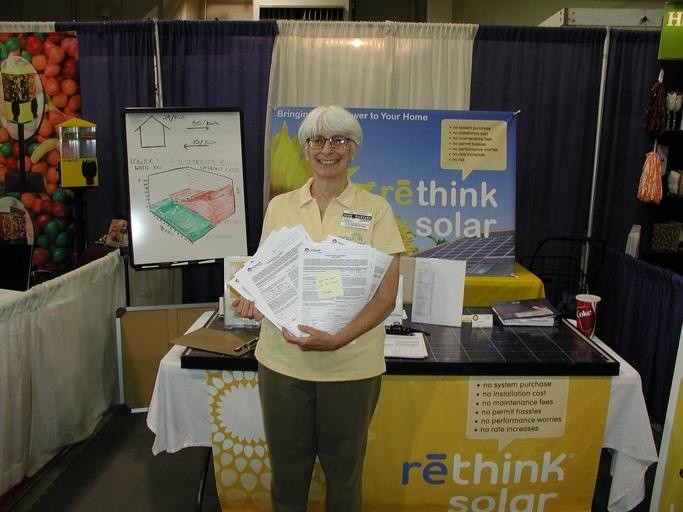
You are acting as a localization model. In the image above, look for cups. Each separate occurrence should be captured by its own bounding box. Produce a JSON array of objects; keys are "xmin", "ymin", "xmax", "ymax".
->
[{"xmin": 574, "ymin": 294, "xmax": 601, "ymax": 338}]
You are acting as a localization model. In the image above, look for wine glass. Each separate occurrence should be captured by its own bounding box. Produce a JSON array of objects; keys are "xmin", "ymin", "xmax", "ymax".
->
[{"xmin": 665, "ymin": 89, "xmax": 683, "ymax": 131}]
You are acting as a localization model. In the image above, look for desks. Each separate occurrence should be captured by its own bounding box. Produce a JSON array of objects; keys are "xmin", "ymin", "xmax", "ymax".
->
[{"xmin": 160, "ymin": 309, "xmax": 642, "ymax": 511}]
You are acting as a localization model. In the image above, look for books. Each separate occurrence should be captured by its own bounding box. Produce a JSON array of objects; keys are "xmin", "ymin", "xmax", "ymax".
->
[
  {"xmin": 221, "ymin": 254, "xmax": 260, "ymax": 330},
  {"xmin": 491, "ymin": 295, "xmax": 558, "ymax": 329}
]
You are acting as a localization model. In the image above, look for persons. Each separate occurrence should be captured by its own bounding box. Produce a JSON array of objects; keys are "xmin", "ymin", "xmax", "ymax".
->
[
  {"xmin": 529, "ymin": 303, "xmax": 553, "ymax": 315},
  {"xmin": 229, "ymin": 103, "xmax": 409, "ymax": 510}
]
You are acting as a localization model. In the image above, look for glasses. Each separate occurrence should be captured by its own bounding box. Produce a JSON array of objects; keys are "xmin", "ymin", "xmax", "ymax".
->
[{"xmin": 304, "ymin": 133, "xmax": 358, "ymax": 149}]
